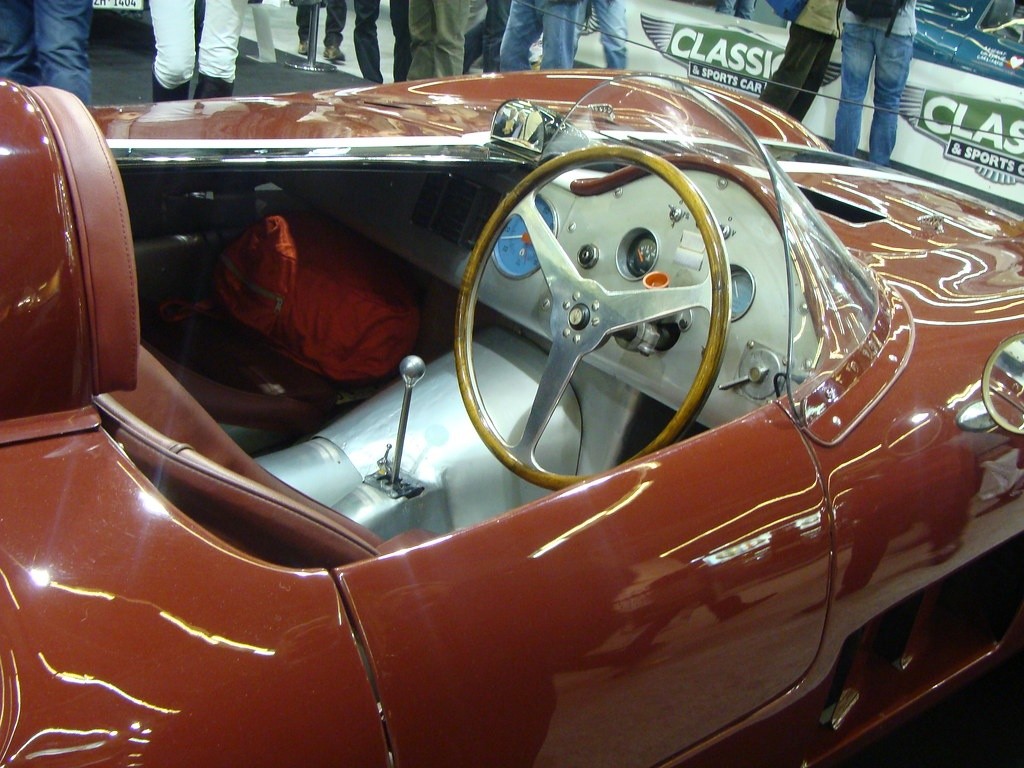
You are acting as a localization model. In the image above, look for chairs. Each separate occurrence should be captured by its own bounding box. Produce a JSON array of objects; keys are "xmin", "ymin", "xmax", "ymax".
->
[{"xmin": 32, "ymin": 86, "xmax": 439, "ymax": 569}]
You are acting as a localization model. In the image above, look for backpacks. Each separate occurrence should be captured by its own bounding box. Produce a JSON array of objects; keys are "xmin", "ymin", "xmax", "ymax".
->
[{"xmin": 846, "ymin": 0, "xmax": 905, "ymax": 37}]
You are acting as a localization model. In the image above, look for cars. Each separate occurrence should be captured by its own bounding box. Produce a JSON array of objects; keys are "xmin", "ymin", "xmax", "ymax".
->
[
  {"xmin": 0, "ymin": 71, "xmax": 1024, "ymax": 765},
  {"xmin": 519, "ymin": 1, "xmax": 1023, "ymax": 216}
]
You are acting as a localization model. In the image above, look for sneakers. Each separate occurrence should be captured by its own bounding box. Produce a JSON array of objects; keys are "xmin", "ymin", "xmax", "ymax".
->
[
  {"xmin": 323, "ymin": 43, "xmax": 344, "ymax": 60},
  {"xmin": 298, "ymin": 40, "xmax": 308, "ymax": 55}
]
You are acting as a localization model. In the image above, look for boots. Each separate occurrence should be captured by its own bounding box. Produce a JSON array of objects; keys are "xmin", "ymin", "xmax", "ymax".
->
[
  {"xmin": 153, "ymin": 61, "xmax": 190, "ymax": 102},
  {"xmin": 193, "ymin": 73, "xmax": 234, "ymax": 99}
]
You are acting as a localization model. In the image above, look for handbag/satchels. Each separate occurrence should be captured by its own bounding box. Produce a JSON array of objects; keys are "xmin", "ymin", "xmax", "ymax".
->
[
  {"xmin": 766, "ymin": 0, "xmax": 808, "ymax": 21},
  {"xmin": 159, "ymin": 207, "xmax": 421, "ymax": 386}
]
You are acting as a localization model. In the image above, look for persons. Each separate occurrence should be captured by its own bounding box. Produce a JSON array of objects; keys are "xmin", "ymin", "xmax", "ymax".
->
[
  {"xmin": 296, "ymin": 0, "xmax": 347, "ymax": 60},
  {"xmin": 715, "ymin": 0, "xmax": 757, "ymax": 21},
  {"xmin": 0, "ymin": 0, "xmax": 95, "ymax": 108},
  {"xmin": 149, "ymin": 0, "xmax": 248, "ymax": 104},
  {"xmin": 759, "ymin": 0, "xmax": 843, "ymax": 123},
  {"xmin": 352, "ymin": 0, "xmax": 628, "ymax": 84},
  {"xmin": 832, "ymin": 0, "xmax": 917, "ymax": 168}
]
